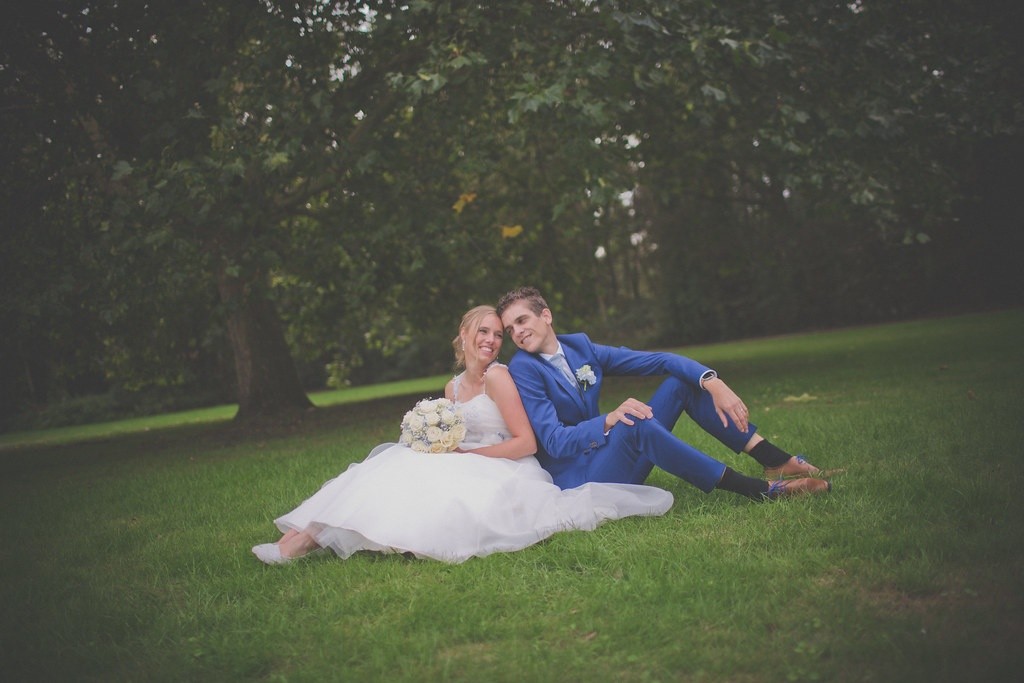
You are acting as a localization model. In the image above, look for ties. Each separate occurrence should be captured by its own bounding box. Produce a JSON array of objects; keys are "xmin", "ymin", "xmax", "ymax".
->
[{"xmin": 550, "ymin": 355, "xmax": 585, "ymax": 403}]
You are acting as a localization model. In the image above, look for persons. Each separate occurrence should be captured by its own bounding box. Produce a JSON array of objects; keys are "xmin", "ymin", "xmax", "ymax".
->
[
  {"xmin": 497, "ymin": 288, "xmax": 844, "ymax": 503},
  {"xmin": 251, "ymin": 305, "xmax": 675, "ymax": 567}
]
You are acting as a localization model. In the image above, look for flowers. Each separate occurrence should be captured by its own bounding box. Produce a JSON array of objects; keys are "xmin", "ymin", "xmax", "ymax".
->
[
  {"xmin": 576, "ymin": 365, "xmax": 596, "ymax": 391},
  {"xmin": 400, "ymin": 396, "xmax": 468, "ymax": 453}
]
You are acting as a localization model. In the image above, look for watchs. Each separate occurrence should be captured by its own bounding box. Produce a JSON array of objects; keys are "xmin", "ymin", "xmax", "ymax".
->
[{"xmin": 702, "ymin": 374, "xmax": 714, "ymax": 382}]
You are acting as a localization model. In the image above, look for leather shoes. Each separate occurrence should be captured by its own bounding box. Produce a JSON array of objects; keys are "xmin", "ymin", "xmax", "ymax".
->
[
  {"xmin": 763, "ymin": 456, "xmax": 846, "ymax": 478},
  {"xmin": 765, "ymin": 478, "xmax": 830, "ymax": 498},
  {"xmin": 252, "ymin": 542, "xmax": 328, "ymax": 565}
]
